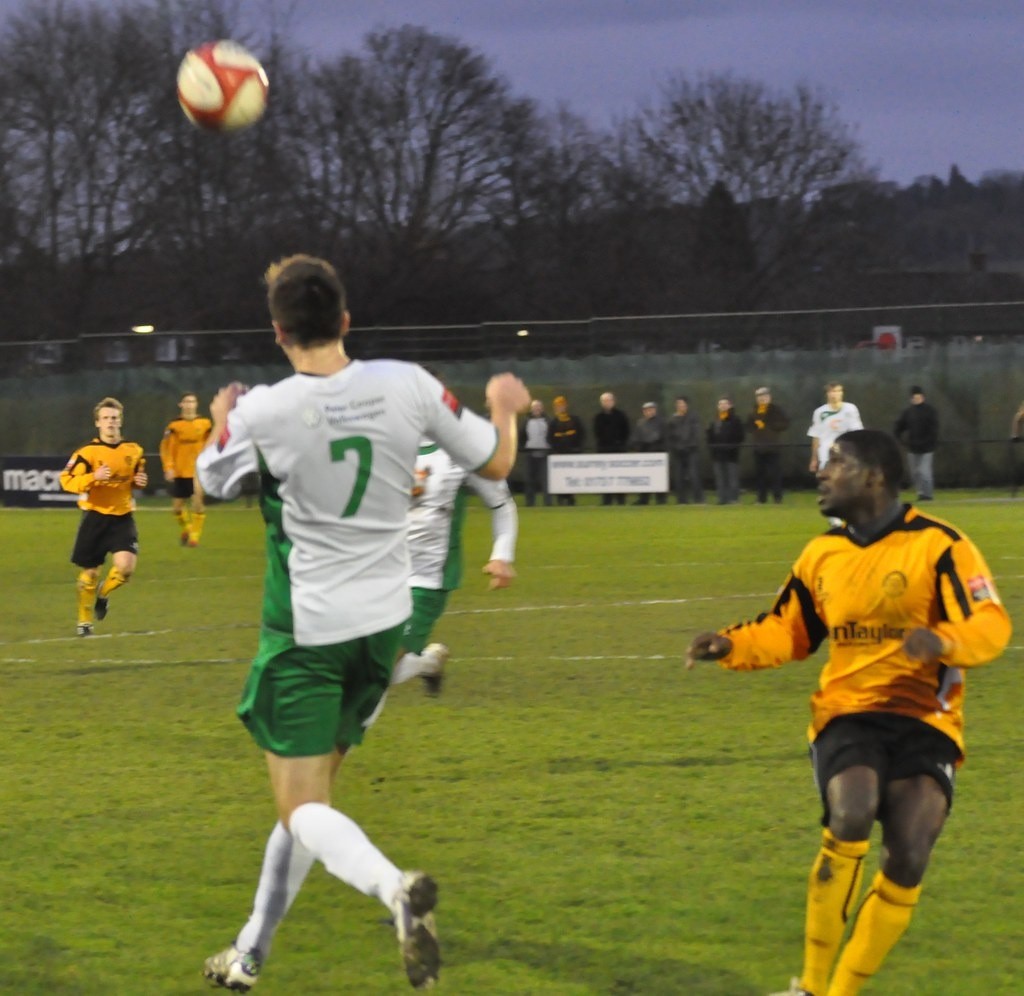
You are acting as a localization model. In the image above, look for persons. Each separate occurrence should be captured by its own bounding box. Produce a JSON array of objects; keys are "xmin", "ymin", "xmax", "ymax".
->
[
  {"xmin": 705, "ymin": 397, "xmax": 744, "ymax": 504},
  {"xmin": 193, "ymin": 256, "xmax": 528, "ymax": 996},
  {"xmin": 393, "ymin": 366, "xmax": 518, "ymax": 696},
  {"xmin": 521, "ymin": 396, "xmax": 584, "ymax": 506},
  {"xmin": 59, "ymin": 398, "xmax": 148, "ymax": 636},
  {"xmin": 808, "ymin": 383, "xmax": 863, "ymax": 529},
  {"xmin": 161, "ymin": 393, "xmax": 213, "ymax": 545},
  {"xmin": 687, "ymin": 429, "xmax": 1011, "ymax": 996},
  {"xmin": 635, "ymin": 398, "xmax": 702, "ymax": 505},
  {"xmin": 744, "ymin": 387, "xmax": 791, "ymax": 504},
  {"xmin": 895, "ymin": 386, "xmax": 938, "ymax": 502},
  {"xmin": 593, "ymin": 392, "xmax": 630, "ymax": 504}
]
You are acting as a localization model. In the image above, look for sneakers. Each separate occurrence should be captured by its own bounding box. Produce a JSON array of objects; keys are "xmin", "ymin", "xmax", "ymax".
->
[
  {"xmin": 94, "ymin": 581, "xmax": 108, "ymax": 621},
  {"xmin": 76, "ymin": 622, "xmax": 95, "ymax": 639},
  {"xmin": 391, "ymin": 871, "xmax": 441, "ymax": 994},
  {"xmin": 202, "ymin": 941, "xmax": 264, "ymax": 995},
  {"xmin": 182, "ymin": 530, "xmax": 200, "ymax": 547},
  {"xmin": 421, "ymin": 642, "xmax": 449, "ymax": 698}
]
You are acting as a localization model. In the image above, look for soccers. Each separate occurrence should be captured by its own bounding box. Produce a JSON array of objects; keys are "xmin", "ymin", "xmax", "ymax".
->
[{"xmin": 175, "ymin": 42, "xmax": 271, "ymax": 131}]
